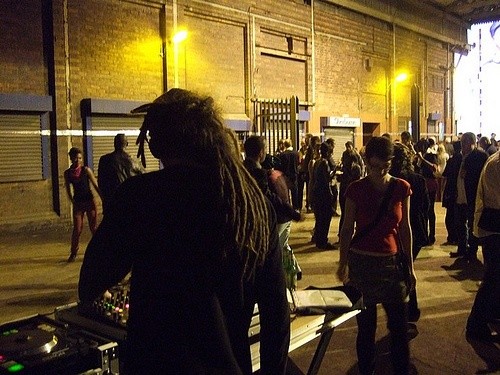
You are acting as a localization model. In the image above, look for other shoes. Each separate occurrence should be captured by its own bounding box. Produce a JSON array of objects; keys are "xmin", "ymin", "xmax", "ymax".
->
[
  {"xmin": 311, "ymin": 236, "xmax": 316, "ymax": 242},
  {"xmin": 450, "ymin": 252, "xmax": 460, "ymax": 257},
  {"xmin": 317, "ymin": 243, "xmax": 336, "ymax": 250},
  {"xmin": 332, "ymin": 213, "xmax": 339, "ymax": 217},
  {"xmin": 307, "ymin": 206, "xmax": 313, "ymax": 213},
  {"xmin": 68, "ymin": 253, "xmax": 77, "ymax": 262},
  {"xmin": 447, "ymin": 236, "xmax": 454, "ymax": 242}
]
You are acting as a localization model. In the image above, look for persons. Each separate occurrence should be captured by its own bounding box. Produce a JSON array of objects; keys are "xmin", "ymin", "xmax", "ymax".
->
[
  {"xmin": 337, "ymin": 136, "xmax": 415, "ymax": 371},
  {"xmin": 243, "ymin": 133, "xmax": 368, "ymax": 279},
  {"xmin": 64, "ymin": 147, "xmax": 105, "ymax": 262},
  {"xmin": 463, "ymin": 148, "xmax": 500, "ymax": 342},
  {"xmin": 77, "ymin": 87, "xmax": 290, "ymax": 375},
  {"xmin": 98, "ymin": 133, "xmax": 148, "ymax": 214},
  {"xmin": 381, "ymin": 131, "xmax": 499, "ymax": 259}
]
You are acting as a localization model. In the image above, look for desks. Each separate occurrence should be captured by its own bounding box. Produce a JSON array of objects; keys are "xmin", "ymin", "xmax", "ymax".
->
[{"xmin": 55, "ymin": 301, "xmax": 361, "ymax": 375}]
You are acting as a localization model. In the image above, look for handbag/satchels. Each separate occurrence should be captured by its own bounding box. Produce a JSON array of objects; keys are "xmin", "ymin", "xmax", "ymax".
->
[{"xmin": 410, "ymin": 209, "xmax": 430, "ymax": 247}]
